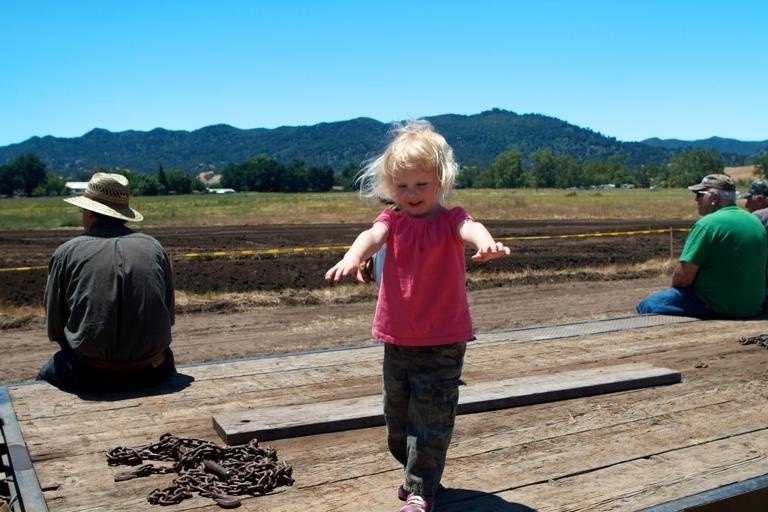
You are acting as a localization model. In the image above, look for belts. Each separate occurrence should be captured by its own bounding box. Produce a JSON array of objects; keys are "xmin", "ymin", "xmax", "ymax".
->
[{"xmin": 71, "ymin": 348, "xmax": 166, "ymax": 371}]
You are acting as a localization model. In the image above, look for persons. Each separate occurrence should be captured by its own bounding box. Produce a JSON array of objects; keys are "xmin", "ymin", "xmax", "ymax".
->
[
  {"xmin": 32, "ymin": 170, "xmax": 176, "ymax": 397},
  {"xmin": 323, "ymin": 117, "xmax": 512, "ymax": 512},
  {"xmin": 735, "ymin": 178, "xmax": 767, "ymax": 233},
  {"xmin": 635, "ymin": 173, "xmax": 767, "ymax": 317}
]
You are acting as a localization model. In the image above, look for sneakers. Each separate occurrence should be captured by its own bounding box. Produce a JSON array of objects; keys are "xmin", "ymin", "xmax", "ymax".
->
[{"xmin": 398, "ymin": 483, "xmax": 435, "ymax": 511}]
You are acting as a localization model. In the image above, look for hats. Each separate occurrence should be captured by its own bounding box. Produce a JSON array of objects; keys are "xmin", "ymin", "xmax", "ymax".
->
[
  {"xmin": 62, "ymin": 171, "xmax": 146, "ymax": 224},
  {"xmin": 736, "ymin": 179, "xmax": 768, "ymax": 201},
  {"xmin": 687, "ymin": 172, "xmax": 737, "ymax": 194}
]
